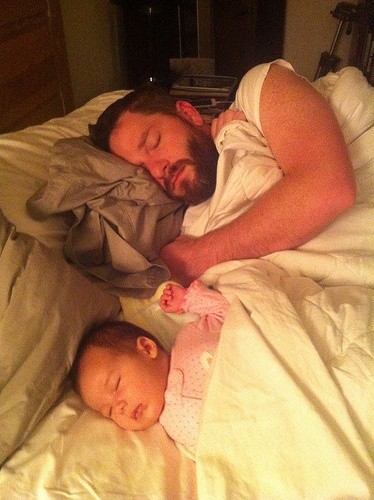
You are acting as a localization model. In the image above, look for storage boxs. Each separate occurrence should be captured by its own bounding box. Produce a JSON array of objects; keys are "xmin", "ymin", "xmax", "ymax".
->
[{"xmin": 171, "ymin": 75, "xmax": 237, "ymax": 107}]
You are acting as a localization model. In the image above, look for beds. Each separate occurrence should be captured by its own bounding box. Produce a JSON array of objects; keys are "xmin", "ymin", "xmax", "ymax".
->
[{"xmin": 0, "ymin": 66, "xmax": 374, "ymax": 500}]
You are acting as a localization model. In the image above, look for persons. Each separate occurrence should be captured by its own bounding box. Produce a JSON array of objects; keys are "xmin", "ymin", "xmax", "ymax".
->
[
  {"xmin": 88, "ymin": 58, "xmax": 357, "ymax": 287},
  {"xmin": 71, "ymin": 280, "xmax": 231, "ymax": 454}
]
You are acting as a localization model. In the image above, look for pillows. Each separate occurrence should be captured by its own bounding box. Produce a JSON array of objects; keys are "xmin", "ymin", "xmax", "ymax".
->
[{"xmin": 0, "ymin": 90, "xmax": 133, "ymax": 469}]
